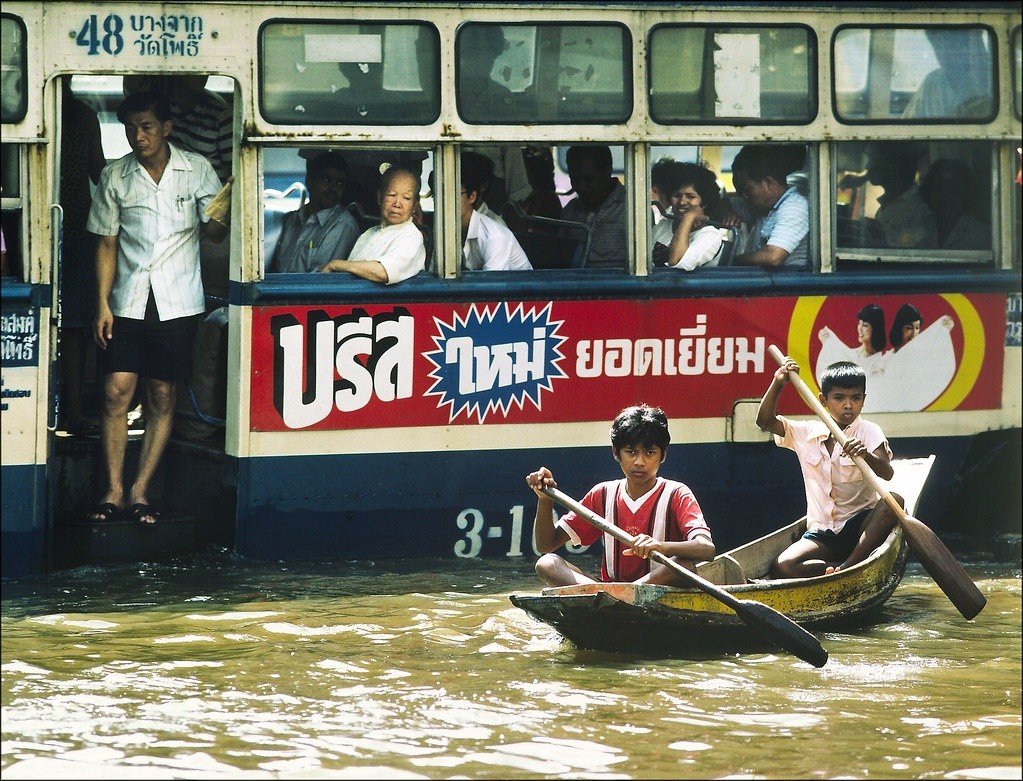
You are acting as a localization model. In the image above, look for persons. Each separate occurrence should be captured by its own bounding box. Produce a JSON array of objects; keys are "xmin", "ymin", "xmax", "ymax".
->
[
  {"xmin": 266, "ymin": 152, "xmax": 426, "ymax": 285},
  {"xmin": 526, "ymin": 405, "xmax": 715, "ymax": 588},
  {"xmin": 428, "ymin": 146, "xmax": 626, "ymax": 270},
  {"xmin": 650, "ymin": 146, "xmax": 810, "ymax": 271},
  {"xmin": 61, "ymin": 73, "xmax": 234, "ymax": 525},
  {"xmin": 818, "ymin": 301, "xmax": 954, "ymax": 378},
  {"xmin": 755, "ymin": 356, "xmax": 905, "ymax": 580},
  {"xmin": 864, "ymin": 140, "xmax": 990, "ymax": 250}
]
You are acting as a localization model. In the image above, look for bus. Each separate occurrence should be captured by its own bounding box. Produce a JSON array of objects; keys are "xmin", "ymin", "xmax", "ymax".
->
[{"xmin": 3, "ymin": 0, "xmax": 1019, "ymax": 539}]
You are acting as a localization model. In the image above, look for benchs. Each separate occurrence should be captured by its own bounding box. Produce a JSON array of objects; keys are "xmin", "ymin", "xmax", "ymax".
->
[
  {"xmin": 348, "ymin": 201, "xmax": 434, "ymax": 271},
  {"xmin": 650, "ymin": 200, "xmax": 740, "ymax": 266},
  {"xmin": 500, "ymin": 200, "xmax": 593, "ymax": 268},
  {"xmin": 838, "ymin": 216, "xmax": 887, "ymax": 248}
]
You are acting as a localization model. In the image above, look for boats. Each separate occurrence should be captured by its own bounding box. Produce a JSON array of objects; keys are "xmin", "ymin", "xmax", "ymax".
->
[{"xmin": 499, "ymin": 452, "xmax": 939, "ymax": 657}]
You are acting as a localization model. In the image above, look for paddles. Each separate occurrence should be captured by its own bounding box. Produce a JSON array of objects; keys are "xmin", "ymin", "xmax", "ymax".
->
[
  {"xmin": 766, "ymin": 343, "xmax": 988, "ymax": 620},
  {"xmin": 538, "ymin": 483, "xmax": 828, "ymax": 668}
]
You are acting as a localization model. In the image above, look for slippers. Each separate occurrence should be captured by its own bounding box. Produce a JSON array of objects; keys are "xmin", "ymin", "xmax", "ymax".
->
[
  {"xmin": 83, "ymin": 502, "xmax": 128, "ymax": 523},
  {"xmin": 126, "ymin": 504, "xmax": 161, "ymax": 526}
]
